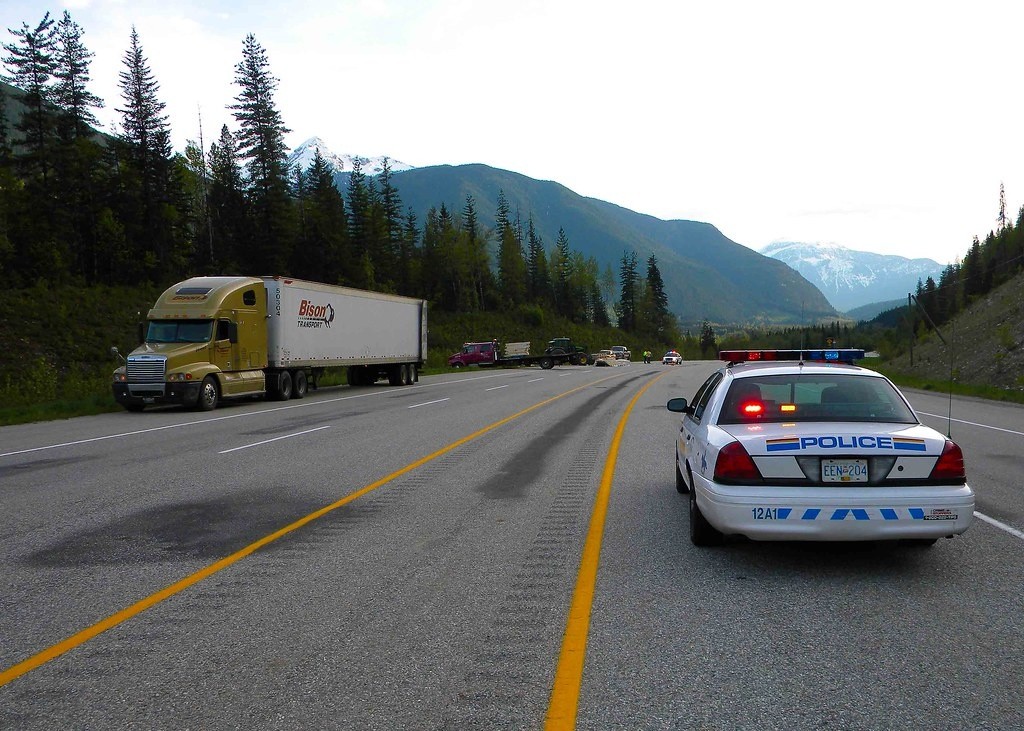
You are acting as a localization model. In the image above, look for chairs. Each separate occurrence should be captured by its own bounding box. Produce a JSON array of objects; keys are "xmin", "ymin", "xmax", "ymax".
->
[{"xmin": 820, "ymin": 386, "xmax": 845, "ymax": 403}]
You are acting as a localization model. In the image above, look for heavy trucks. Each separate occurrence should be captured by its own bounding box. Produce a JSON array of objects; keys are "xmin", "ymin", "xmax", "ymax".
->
[
  {"xmin": 448, "ymin": 338, "xmax": 569, "ymax": 369},
  {"xmin": 111, "ymin": 275, "xmax": 426, "ymax": 412}
]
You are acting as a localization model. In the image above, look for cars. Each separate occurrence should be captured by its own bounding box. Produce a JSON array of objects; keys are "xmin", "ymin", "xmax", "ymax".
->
[
  {"xmin": 596, "ymin": 338, "xmax": 632, "ymax": 360},
  {"xmin": 662, "ymin": 350, "xmax": 682, "ymax": 364},
  {"xmin": 666, "ymin": 349, "xmax": 975, "ymax": 547}
]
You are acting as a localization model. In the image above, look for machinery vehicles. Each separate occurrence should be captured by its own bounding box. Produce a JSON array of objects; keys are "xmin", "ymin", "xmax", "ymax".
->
[{"xmin": 544, "ymin": 337, "xmax": 591, "ymax": 366}]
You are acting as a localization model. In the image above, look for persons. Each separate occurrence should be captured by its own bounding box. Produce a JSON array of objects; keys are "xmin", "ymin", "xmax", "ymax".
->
[
  {"xmin": 647, "ymin": 350, "xmax": 652, "ymax": 364},
  {"xmin": 643, "ymin": 350, "xmax": 648, "ymax": 364}
]
